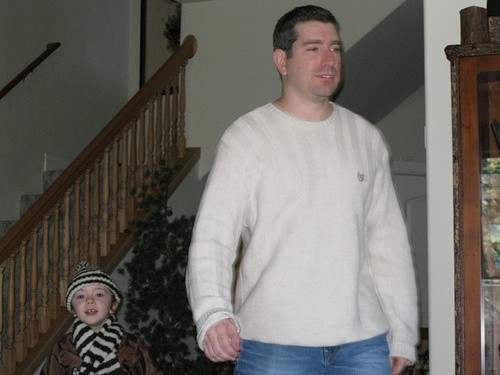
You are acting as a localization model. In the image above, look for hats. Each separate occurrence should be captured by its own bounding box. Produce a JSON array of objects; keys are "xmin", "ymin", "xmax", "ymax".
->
[{"xmin": 66, "ymin": 261, "xmax": 122, "ymax": 314}]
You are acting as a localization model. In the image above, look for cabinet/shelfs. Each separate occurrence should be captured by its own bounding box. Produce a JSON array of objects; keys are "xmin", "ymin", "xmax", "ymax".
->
[{"xmin": 445, "ymin": 42, "xmax": 500, "ymax": 374}]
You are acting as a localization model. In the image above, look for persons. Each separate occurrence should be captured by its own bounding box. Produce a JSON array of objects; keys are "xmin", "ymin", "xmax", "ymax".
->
[
  {"xmin": 185, "ymin": 4, "xmax": 419, "ymax": 375},
  {"xmin": 46, "ymin": 262, "xmax": 164, "ymax": 375}
]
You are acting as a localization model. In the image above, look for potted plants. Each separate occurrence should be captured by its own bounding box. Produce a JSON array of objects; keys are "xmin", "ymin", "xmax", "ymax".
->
[{"xmin": 118, "ymin": 168, "xmax": 236, "ymax": 375}]
